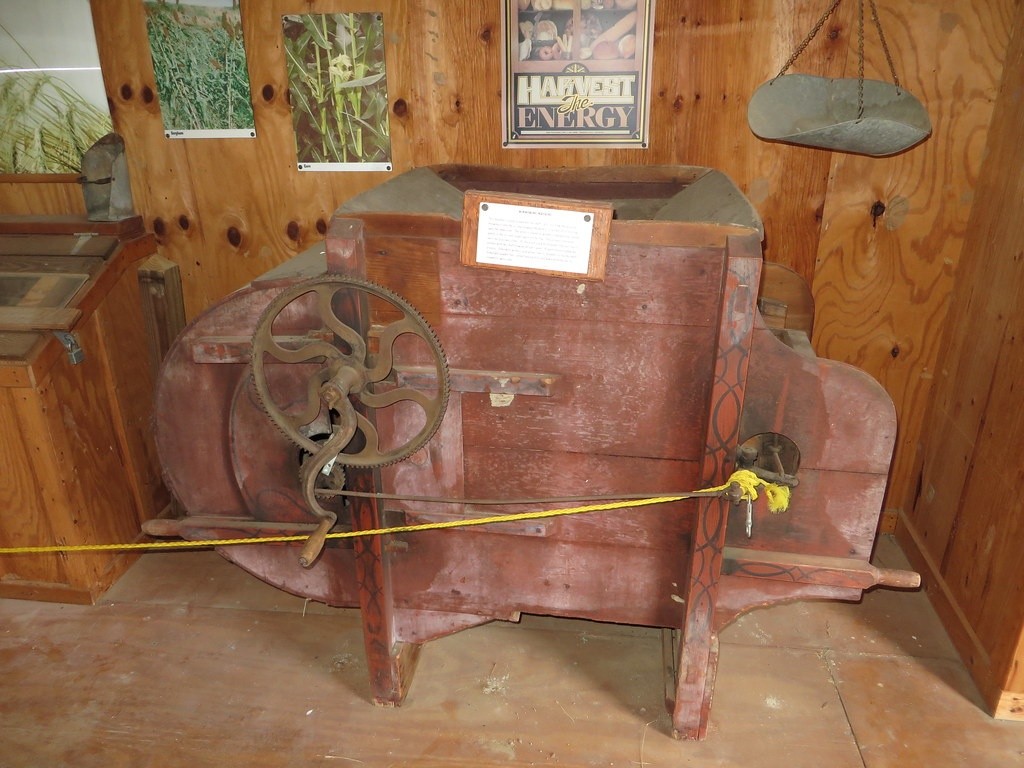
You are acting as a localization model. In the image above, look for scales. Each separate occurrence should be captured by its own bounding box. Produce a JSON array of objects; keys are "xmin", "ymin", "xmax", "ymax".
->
[{"xmin": 745, "ymin": 1, "xmax": 932, "ymax": 157}]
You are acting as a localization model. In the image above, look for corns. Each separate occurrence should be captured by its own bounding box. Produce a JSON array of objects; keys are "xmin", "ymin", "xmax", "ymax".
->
[{"xmin": 334, "ymin": 55, "xmax": 345, "ymax": 105}]
